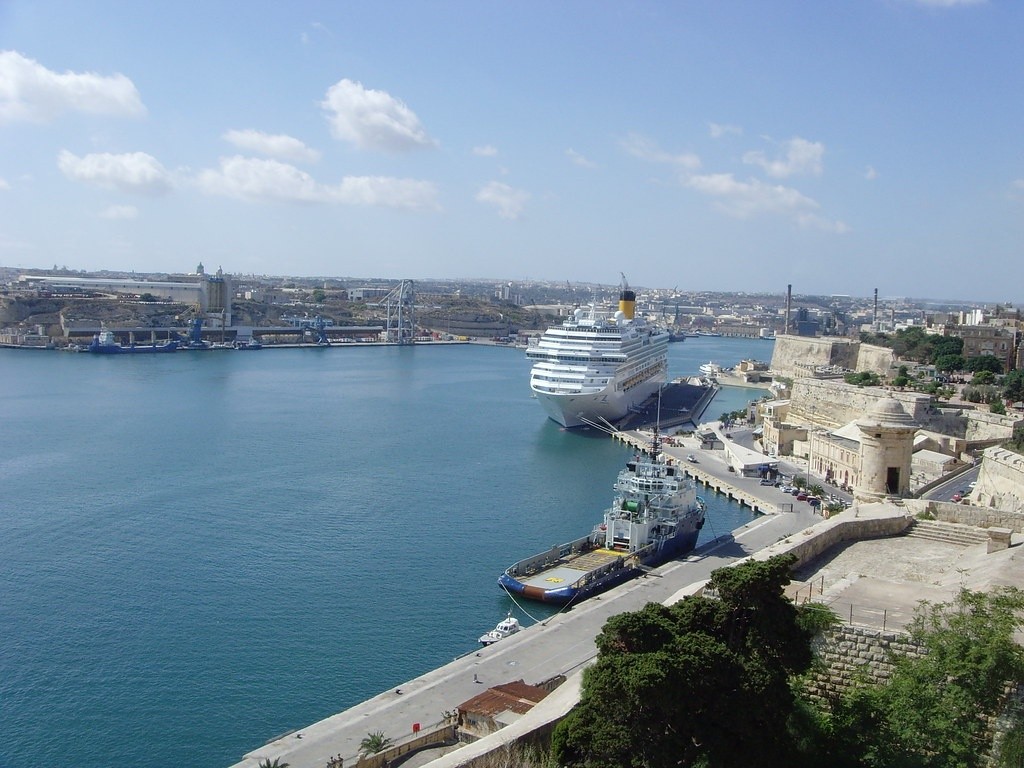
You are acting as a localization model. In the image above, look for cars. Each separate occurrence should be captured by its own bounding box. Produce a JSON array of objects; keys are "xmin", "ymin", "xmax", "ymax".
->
[
  {"xmin": 687, "ymin": 455, "xmax": 697, "ymax": 462},
  {"xmin": 759, "ymin": 479, "xmax": 852, "ymax": 508},
  {"xmin": 660, "ymin": 434, "xmax": 684, "ymax": 447},
  {"xmin": 950, "ymin": 482, "xmax": 977, "ymax": 503}
]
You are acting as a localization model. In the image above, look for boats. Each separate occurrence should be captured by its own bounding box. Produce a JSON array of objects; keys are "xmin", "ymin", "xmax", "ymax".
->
[
  {"xmin": 237, "ymin": 340, "xmax": 262, "ymax": 350},
  {"xmin": 497, "ymin": 382, "xmax": 708, "ymax": 603},
  {"xmin": 478, "ymin": 613, "xmax": 525, "ymax": 646},
  {"xmin": 87, "ymin": 320, "xmax": 176, "ymax": 354},
  {"xmin": 669, "ymin": 326, "xmax": 686, "ymax": 342}
]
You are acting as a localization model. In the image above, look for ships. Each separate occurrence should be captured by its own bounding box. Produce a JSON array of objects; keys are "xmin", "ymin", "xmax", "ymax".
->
[{"xmin": 524, "ymin": 287, "xmax": 670, "ymax": 427}]
